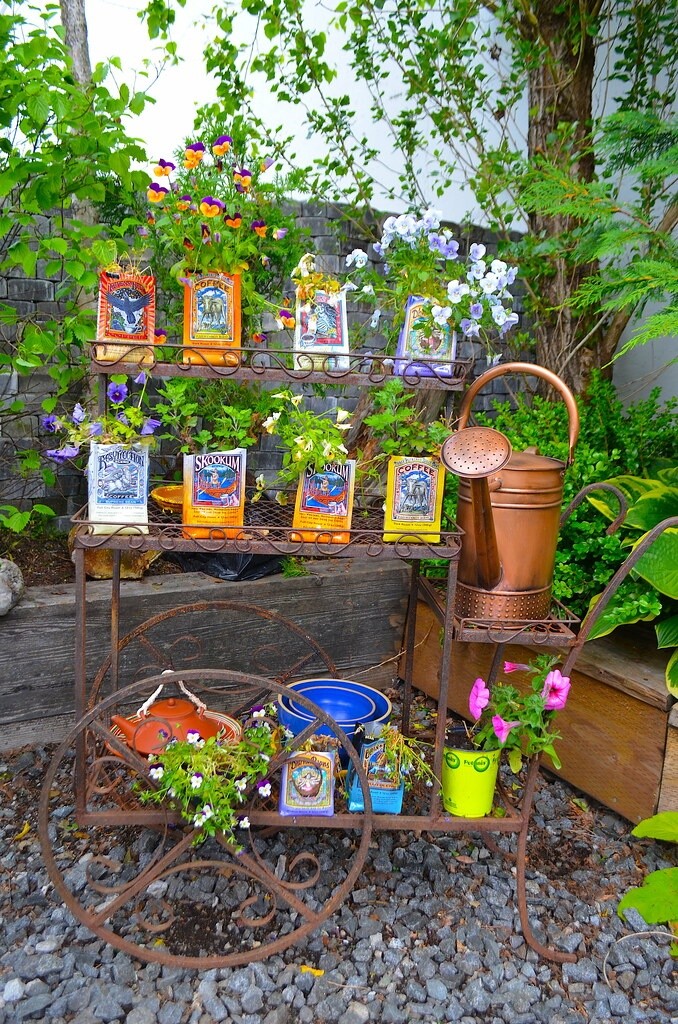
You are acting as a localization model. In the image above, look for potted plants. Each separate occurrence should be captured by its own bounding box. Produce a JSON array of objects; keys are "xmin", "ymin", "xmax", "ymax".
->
[
  {"xmin": 395, "ymin": 364, "xmax": 678, "ymax": 825},
  {"xmin": 0, "ymin": 377, "xmax": 413, "ymax": 726}
]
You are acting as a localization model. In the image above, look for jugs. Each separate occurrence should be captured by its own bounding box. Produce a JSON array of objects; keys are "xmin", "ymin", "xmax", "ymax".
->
[{"xmin": 440, "ymin": 361, "xmax": 581, "ymax": 629}]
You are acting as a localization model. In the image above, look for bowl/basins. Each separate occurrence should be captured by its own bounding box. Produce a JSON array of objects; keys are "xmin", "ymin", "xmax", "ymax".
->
[
  {"xmin": 105, "ymin": 706, "xmax": 244, "ymax": 766},
  {"xmin": 150, "ymin": 485, "xmax": 183, "ymax": 513},
  {"xmin": 277, "ymin": 677, "xmax": 393, "ymax": 773},
  {"xmin": 289, "ymin": 686, "xmax": 377, "ymax": 725}
]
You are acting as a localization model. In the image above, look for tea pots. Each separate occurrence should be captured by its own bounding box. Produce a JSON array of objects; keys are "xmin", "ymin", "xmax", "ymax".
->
[{"xmin": 109, "ymin": 668, "xmax": 224, "ymax": 759}]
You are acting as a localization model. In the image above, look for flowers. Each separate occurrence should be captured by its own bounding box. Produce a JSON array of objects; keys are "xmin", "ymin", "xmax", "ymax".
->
[{"xmin": 40, "ymin": 125, "xmax": 569, "ymax": 828}]
